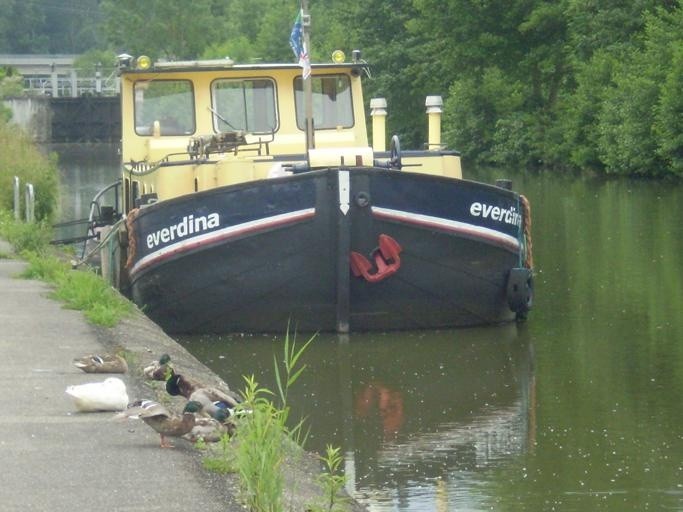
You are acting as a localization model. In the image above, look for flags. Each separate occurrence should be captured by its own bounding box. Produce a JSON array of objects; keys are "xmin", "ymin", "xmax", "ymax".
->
[{"xmin": 286, "ymin": 8, "xmax": 311, "ymax": 81}]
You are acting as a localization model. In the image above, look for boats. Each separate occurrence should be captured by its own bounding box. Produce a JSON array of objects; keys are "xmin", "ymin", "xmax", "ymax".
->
[{"xmin": 81, "ymin": 50, "xmax": 534, "ymax": 336}]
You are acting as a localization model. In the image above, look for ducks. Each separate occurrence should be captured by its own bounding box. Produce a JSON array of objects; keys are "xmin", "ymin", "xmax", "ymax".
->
[
  {"xmin": 127, "ymin": 354, "xmax": 253, "ymax": 448},
  {"xmin": 72, "ymin": 353, "xmax": 129, "ymax": 373},
  {"xmin": 64, "ymin": 377, "xmax": 129, "ymax": 412}
]
